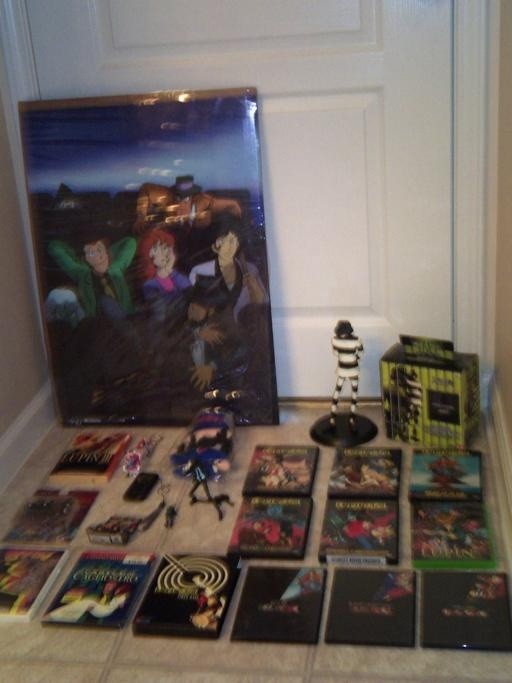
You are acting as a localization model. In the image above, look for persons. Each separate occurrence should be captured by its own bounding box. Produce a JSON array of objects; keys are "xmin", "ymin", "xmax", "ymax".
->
[{"xmin": 329, "ymin": 320, "xmax": 364, "ymax": 431}]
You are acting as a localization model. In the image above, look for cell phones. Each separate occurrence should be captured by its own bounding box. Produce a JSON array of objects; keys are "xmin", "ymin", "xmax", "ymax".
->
[{"xmin": 124, "ymin": 472, "xmax": 159, "ymax": 500}]
